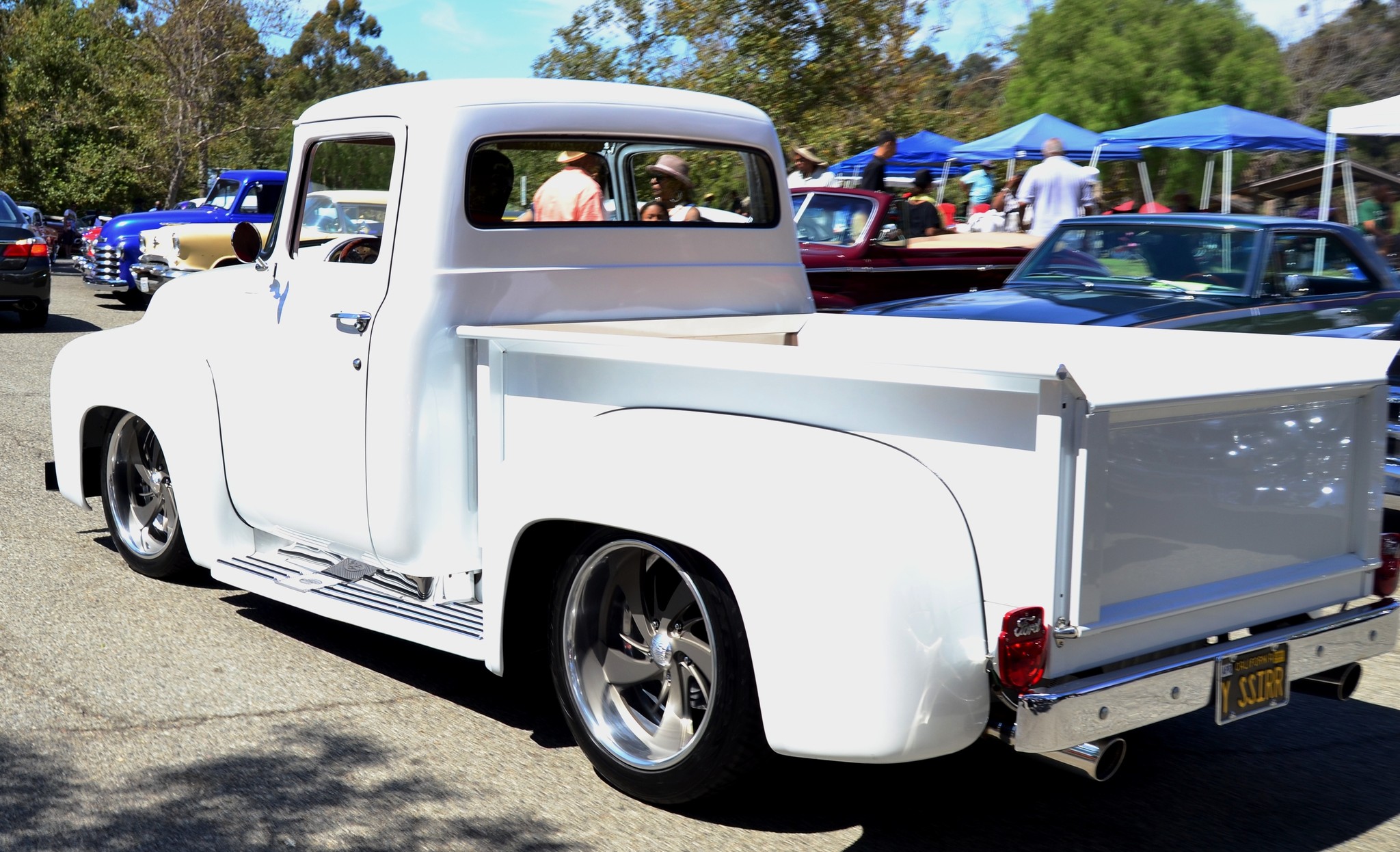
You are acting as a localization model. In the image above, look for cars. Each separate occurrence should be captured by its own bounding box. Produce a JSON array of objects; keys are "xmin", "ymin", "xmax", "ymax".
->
[
  {"xmin": 0, "ymin": 168, "xmax": 388, "ymax": 329},
  {"xmin": 843, "ymin": 212, "xmax": 1399, "ymax": 433}
]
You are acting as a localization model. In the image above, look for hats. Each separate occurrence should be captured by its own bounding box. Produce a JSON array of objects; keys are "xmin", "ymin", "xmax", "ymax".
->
[
  {"xmin": 978, "ymin": 160, "xmax": 996, "ymax": 169},
  {"xmin": 912, "ymin": 169, "xmax": 935, "ymax": 184},
  {"xmin": 647, "ymin": 154, "xmax": 698, "ymax": 189},
  {"xmin": 789, "ymin": 145, "xmax": 825, "ymax": 163},
  {"xmin": 557, "ymin": 151, "xmax": 588, "ymax": 163}
]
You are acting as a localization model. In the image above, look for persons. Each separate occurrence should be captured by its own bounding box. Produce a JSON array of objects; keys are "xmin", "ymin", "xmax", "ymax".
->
[
  {"xmin": 149, "ymin": 200, "xmax": 164, "ymax": 212},
  {"xmin": 1358, "ymin": 177, "xmax": 1396, "ymax": 256},
  {"xmin": 1015, "ymin": 137, "xmax": 1094, "ymax": 240},
  {"xmin": 959, "ymin": 159, "xmax": 997, "ymax": 224},
  {"xmin": 787, "ymin": 144, "xmax": 849, "ymax": 242},
  {"xmin": 64, "ymin": 202, "xmax": 77, "ymax": 259},
  {"xmin": 993, "ymin": 170, "xmax": 1031, "ymax": 229},
  {"xmin": 860, "ymin": 130, "xmax": 897, "ymax": 238},
  {"xmin": 898, "ymin": 169, "xmax": 955, "ymax": 236},
  {"xmin": 645, "ymin": 154, "xmax": 700, "ymax": 221},
  {"xmin": 511, "ymin": 150, "xmax": 609, "ymax": 222},
  {"xmin": 639, "ymin": 201, "xmax": 670, "ymax": 221}
]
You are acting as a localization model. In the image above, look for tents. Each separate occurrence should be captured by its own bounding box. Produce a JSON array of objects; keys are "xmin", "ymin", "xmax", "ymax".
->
[
  {"xmin": 1097, "ymin": 103, "xmax": 1349, "ymax": 273},
  {"xmin": 840, "ymin": 129, "xmax": 971, "ymax": 177},
  {"xmin": 947, "ymin": 111, "xmax": 1143, "ymax": 183},
  {"xmin": 1313, "ymin": 95, "xmax": 1400, "ymax": 276},
  {"xmin": 826, "ymin": 138, "xmax": 905, "ymax": 178}
]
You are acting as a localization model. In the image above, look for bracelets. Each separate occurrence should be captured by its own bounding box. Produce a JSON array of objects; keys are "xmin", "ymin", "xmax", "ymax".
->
[{"xmin": 1000, "ymin": 187, "xmax": 1011, "ymax": 192}]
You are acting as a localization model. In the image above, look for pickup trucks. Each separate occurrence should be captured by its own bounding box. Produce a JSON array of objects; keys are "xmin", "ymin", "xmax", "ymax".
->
[
  {"xmin": 787, "ymin": 170, "xmax": 1101, "ymax": 312},
  {"xmin": 31, "ymin": 75, "xmax": 1399, "ymax": 805}
]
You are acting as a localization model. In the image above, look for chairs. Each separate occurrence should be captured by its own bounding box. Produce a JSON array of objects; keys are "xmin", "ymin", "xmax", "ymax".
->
[{"xmin": 468, "ymin": 150, "xmax": 515, "ymax": 220}]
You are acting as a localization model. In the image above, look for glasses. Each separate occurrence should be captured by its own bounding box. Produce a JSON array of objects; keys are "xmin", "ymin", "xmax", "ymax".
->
[
  {"xmin": 796, "ymin": 158, "xmax": 803, "ymax": 162},
  {"xmin": 650, "ymin": 174, "xmax": 668, "ymax": 180}
]
